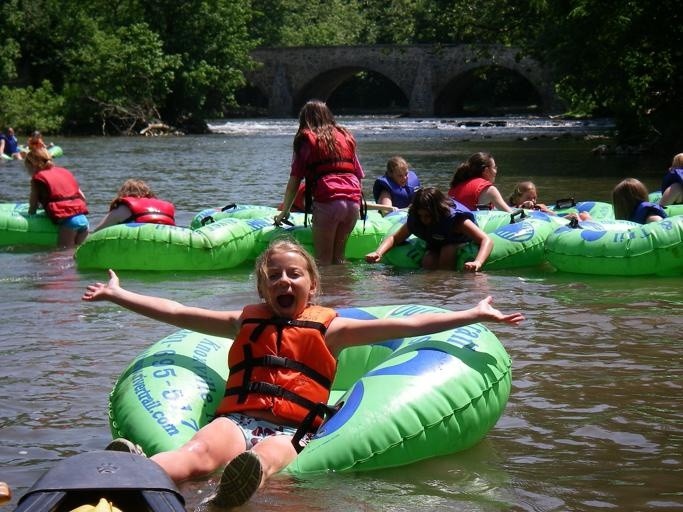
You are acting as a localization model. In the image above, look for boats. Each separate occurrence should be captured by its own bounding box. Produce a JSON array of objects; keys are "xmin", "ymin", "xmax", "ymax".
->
[
  {"xmin": 0, "ymin": 197, "xmax": 59, "ymax": 253},
  {"xmin": 18, "ymin": 144, "xmax": 63, "ymax": 162},
  {"xmin": 96, "ymin": 301, "xmax": 524, "ymax": 486}
]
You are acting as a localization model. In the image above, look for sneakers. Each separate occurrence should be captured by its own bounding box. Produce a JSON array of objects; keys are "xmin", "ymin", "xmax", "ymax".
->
[
  {"xmin": 106, "ymin": 438, "xmax": 145, "ymax": 455},
  {"xmin": 201, "ymin": 451, "xmax": 262, "ymax": 507}
]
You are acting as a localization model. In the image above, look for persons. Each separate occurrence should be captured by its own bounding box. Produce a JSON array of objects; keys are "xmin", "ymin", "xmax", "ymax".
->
[
  {"xmin": 0, "ymin": 127, "xmax": 26, "ymax": 161},
  {"xmin": 610, "ymin": 177, "xmax": 667, "ymax": 224},
  {"xmin": 448, "ymin": 152, "xmax": 513, "ymax": 213},
  {"xmin": 27, "ymin": 130, "xmax": 53, "ymax": 150},
  {"xmin": 660, "ymin": 153, "xmax": 683, "ymax": 206},
  {"xmin": 89, "ymin": 179, "xmax": 176, "ymax": 233},
  {"xmin": 81, "ymin": 240, "xmax": 525, "ymax": 509},
  {"xmin": 373, "ymin": 156, "xmax": 420, "ymax": 218},
  {"xmin": 508, "ymin": 181, "xmax": 591, "ymax": 221},
  {"xmin": 365, "ymin": 187, "xmax": 493, "ymax": 271},
  {"xmin": 278, "ymin": 183, "xmax": 398, "ymax": 217},
  {"xmin": 274, "ymin": 99, "xmax": 365, "ymax": 265},
  {"xmin": 24, "ymin": 149, "xmax": 90, "ymax": 249}
]
